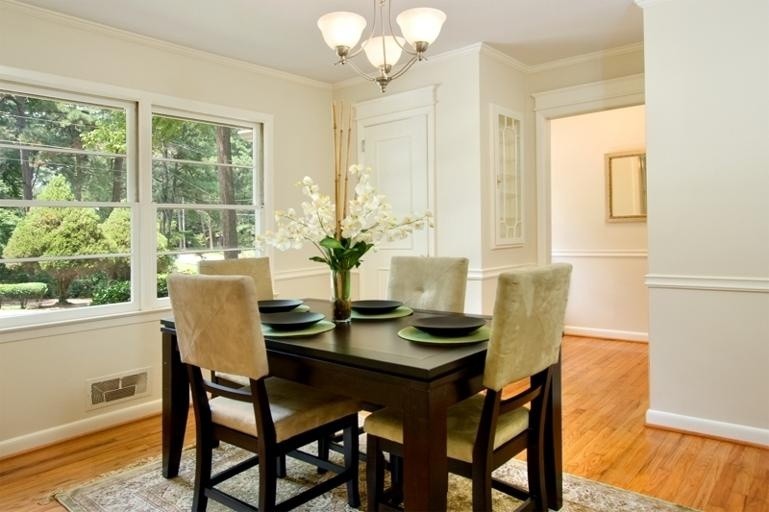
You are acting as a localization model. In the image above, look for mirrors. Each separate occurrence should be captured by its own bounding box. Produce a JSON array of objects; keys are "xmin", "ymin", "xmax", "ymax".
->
[{"xmin": 604, "ymin": 148, "xmax": 648, "ymax": 222}]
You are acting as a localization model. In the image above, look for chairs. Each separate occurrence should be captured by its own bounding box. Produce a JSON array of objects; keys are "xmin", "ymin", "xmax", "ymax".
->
[
  {"xmin": 197, "ymin": 257, "xmax": 276, "ymax": 304},
  {"xmin": 362, "ymin": 262, "xmax": 573, "ymax": 512},
  {"xmin": 163, "ymin": 271, "xmax": 361, "ymax": 512},
  {"xmin": 386, "ymin": 253, "xmax": 469, "ymax": 315}
]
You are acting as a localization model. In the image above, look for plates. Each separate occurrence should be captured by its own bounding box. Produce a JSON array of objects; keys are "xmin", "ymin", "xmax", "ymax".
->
[
  {"xmin": 260, "ymin": 320, "xmax": 337, "ymax": 338},
  {"xmin": 258, "ymin": 299, "xmax": 305, "ymax": 311},
  {"xmin": 350, "ymin": 306, "xmax": 415, "ymax": 320},
  {"xmin": 396, "ymin": 326, "xmax": 492, "ymax": 344},
  {"xmin": 260, "ymin": 311, "xmax": 327, "ymax": 332},
  {"xmin": 409, "ymin": 316, "xmax": 487, "ymax": 337},
  {"xmin": 294, "ymin": 304, "xmax": 311, "ymax": 312}
]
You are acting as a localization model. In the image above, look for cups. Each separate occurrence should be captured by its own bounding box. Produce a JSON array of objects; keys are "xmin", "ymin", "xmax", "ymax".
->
[{"xmin": 351, "ymin": 299, "xmax": 403, "ymax": 315}]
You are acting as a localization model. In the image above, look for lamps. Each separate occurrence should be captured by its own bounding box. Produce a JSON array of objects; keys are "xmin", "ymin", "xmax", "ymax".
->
[{"xmin": 317, "ymin": 0, "xmax": 449, "ymax": 96}]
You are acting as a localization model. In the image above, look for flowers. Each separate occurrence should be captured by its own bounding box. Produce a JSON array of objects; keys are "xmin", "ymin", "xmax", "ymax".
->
[{"xmin": 265, "ymin": 162, "xmax": 439, "ymax": 270}]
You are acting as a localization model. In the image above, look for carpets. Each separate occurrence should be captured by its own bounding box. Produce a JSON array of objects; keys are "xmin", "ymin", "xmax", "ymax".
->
[{"xmin": 51, "ymin": 413, "xmax": 704, "ymax": 512}]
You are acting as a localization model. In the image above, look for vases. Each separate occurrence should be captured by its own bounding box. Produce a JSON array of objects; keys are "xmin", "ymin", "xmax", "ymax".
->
[{"xmin": 331, "ymin": 268, "xmax": 356, "ymax": 325}]
